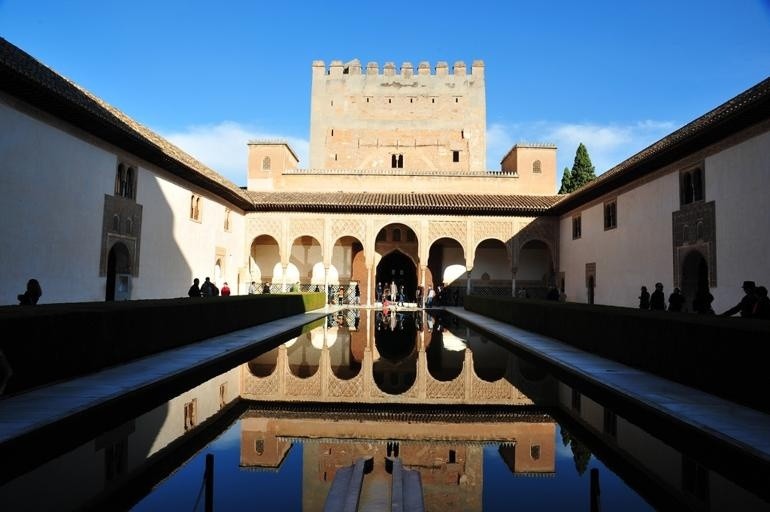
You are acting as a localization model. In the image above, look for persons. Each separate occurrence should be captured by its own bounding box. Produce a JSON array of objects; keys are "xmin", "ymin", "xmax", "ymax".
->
[
  {"xmin": 518, "ymin": 287, "xmax": 528, "ymax": 299},
  {"xmin": 262, "ymin": 282, "xmax": 270, "ymax": 294},
  {"xmin": 377, "ymin": 281, "xmax": 464, "ymax": 308},
  {"xmin": 314, "ymin": 280, "xmax": 361, "ymax": 306},
  {"xmin": 18, "ymin": 279, "xmax": 42, "ymax": 306},
  {"xmin": 545, "ymin": 287, "xmax": 567, "ymax": 302},
  {"xmin": 328, "ymin": 307, "xmax": 444, "ymax": 332},
  {"xmin": 249, "ymin": 281, "xmax": 256, "ymax": 294},
  {"xmin": 188, "ymin": 278, "xmax": 201, "ymax": 297},
  {"xmin": 201, "ymin": 277, "xmax": 219, "ymax": 297},
  {"xmin": 221, "ymin": 282, "xmax": 230, "ymax": 297},
  {"xmin": 290, "ymin": 282, "xmax": 300, "ymax": 293},
  {"xmin": 638, "ymin": 281, "xmax": 770, "ymax": 320}
]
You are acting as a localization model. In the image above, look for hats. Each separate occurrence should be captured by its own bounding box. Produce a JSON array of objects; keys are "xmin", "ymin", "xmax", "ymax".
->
[
  {"xmin": 741, "ymin": 281, "xmax": 756, "ymax": 289},
  {"xmin": 755, "ymin": 287, "xmax": 768, "ymax": 299}
]
